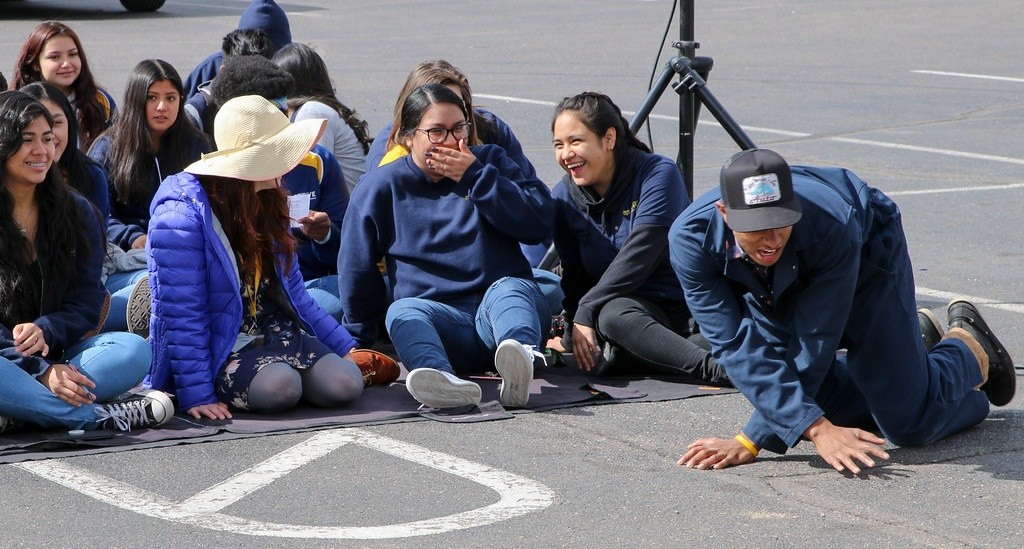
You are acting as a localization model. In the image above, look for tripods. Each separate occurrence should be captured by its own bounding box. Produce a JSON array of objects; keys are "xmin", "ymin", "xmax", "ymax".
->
[{"xmin": 538, "ymin": 0, "xmax": 757, "ymax": 270}]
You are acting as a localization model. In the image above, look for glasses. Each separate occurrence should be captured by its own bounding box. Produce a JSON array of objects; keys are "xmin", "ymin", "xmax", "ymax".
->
[{"xmin": 413, "ymin": 124, "xmax": 472, "ymax": 145}]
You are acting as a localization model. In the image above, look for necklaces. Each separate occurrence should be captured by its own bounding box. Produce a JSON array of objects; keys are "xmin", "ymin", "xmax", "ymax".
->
[{"xmin": 12, "ymin": 207, "xmax": 33, "ymax": 235}]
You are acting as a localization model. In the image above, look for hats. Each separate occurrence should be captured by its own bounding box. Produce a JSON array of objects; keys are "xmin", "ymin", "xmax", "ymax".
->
[
  {"xmin": 181, "ymin": 95, "xmax": 327, "ymax": 181},
  {"xmin": 719, "ymin": 148, "xmax": 801, "ymax": 232},
  {"xmin": 238, "ymin": 0, "xmax": 292, "ymax": 53}
]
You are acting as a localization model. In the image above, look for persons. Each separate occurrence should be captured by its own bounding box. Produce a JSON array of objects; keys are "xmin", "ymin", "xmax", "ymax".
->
[
  {"xmin": 337, "ymin": 82, "xmax": 558, "ymax": 409},
  {"xmin": 9, "ymin": 21, "xmax": 119, "ymax": 157},
  {"xmin": 209, "ymin": 54, "xmax": 365, "ymax": 196},
  {"xmin": 271, "ymin": 43, "xmax": 336, "ymax": 100},
  {"xmin": 521, "ymin": 91, "xmax": 735, "ymax": 386},
  {"xmin": 365, "ymin": 60, "xmax": 552, "ymax": 195},
  {"xmin": 182, "ymin": 0, "xmax": 292, "ymax": 102},
  {"xmin": 183, "ymin": 27, "xmax": 276, "ymax": 152},
  {"xmin": 0, "ymin": 90, "xmax": 175, "ymax": 431},
  {"xmin": 140, "ymin": 94, "xmax": 401, "ymax": 419},
  {"xmin": 280, "ymin": 144, "xmax": 349, "ymax": 282},
  {"xmin": 17, "ymin": 81, "xmax": 150, "ymax": 339},
  {"xmin": 85, "ymin": 59, "xmax": 212, "ymax": 250},
  {"xmin": 668, "ymin": 147, "xmax": 1017, "ymax": 474}
]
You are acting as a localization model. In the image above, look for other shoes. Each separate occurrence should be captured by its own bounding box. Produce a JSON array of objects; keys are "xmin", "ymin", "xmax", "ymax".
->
[
  {"xmin": 916, "ymin": 308, "xmax": 946, "ymax": 352},
  {"xmin": 84, "ymin": 289, "xmax": 112, "ymax": 340},
  {"xmin": 947, "ymin": 298, "xmax": 1016, "ymax": 407},
  {"xmin": 407, "ymin": 368, "xmax": 482, "ymax": 410},
  {"xmin": 126, "ymin": 275, "xmax": 153, "ymax": 337},
  {"xmin": 349, "ymin": 348, "xmax": 400, "ymax": 388},
  {"xmin": 494, "ymin": 337, "xmax": 548, "ymax": 408}
]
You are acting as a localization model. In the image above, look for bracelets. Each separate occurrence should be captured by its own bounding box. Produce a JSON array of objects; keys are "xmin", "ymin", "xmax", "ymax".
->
[{"xmin": 735, "ymin": 434, "xmax": 758, "ymax": 457}]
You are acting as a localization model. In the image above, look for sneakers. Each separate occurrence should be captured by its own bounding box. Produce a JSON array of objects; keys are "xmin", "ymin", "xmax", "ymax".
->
[{"xmin": 92, "ymin": 389, "xmax": 175, "ymax": 433}]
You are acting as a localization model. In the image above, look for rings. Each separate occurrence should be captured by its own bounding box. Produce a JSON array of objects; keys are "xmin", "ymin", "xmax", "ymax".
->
[{"xmin": 35, "ymin": 334, "xmax": 39, "ymax": 340}]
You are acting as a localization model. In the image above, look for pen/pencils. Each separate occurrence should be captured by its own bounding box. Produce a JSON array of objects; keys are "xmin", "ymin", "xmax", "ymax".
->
[{"xmin": 67, "ymin": 360, "xmax": 93, "ymax": 401}]
